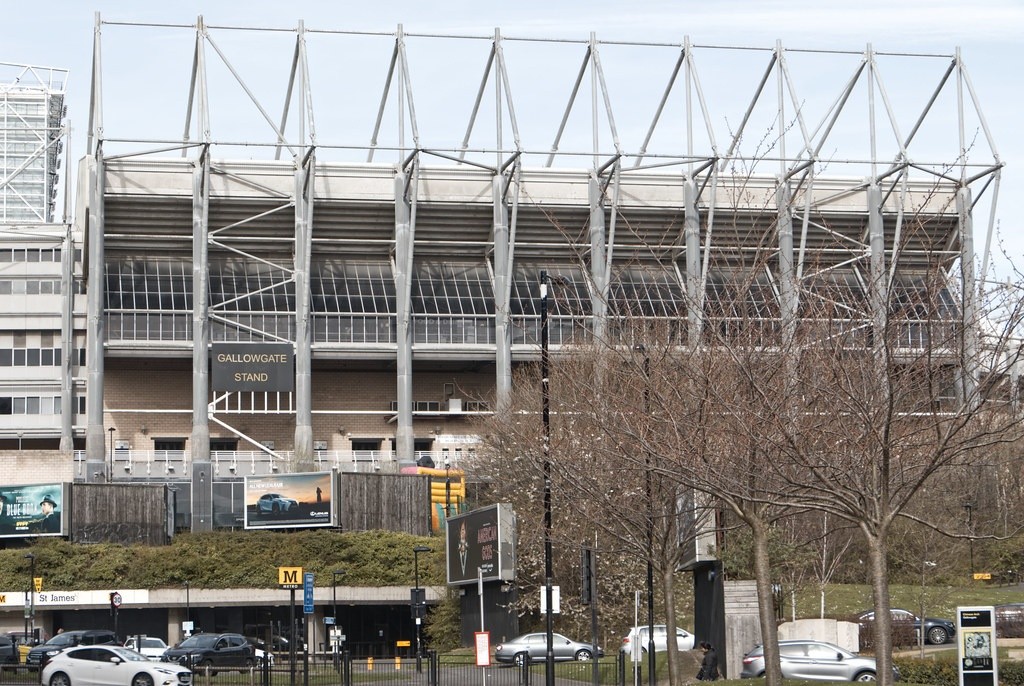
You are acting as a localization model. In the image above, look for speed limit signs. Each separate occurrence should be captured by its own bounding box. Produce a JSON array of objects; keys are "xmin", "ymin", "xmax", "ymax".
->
[{"xmin": 112, "ymin": 594, "xmax": 122, "ymax": 607}]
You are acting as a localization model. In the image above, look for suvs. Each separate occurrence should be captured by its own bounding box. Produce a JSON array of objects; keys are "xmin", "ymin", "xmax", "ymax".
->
[
  {"xmin": 740, "ymin": 639, "xmax": 900, "ymax": 682},
  {"xmin": 619, "ymin": 625, "xmax": 695, "ymax": 654},
  {"xmin": 26, "ymin": 630, "xmax": 122, "ymax": 671},
  {"xmin": 160, "ymin": 633, "xmax": 257, "ymax": 679}
]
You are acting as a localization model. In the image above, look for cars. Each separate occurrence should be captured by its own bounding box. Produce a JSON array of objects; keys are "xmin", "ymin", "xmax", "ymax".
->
[
  {"xmin": 494, "ymin": 632, "xmax": 603, "ymax": 667},
  {"xmin": 41, "ymin": 644, "xmax": 193, "ymax": 686},
  {"xmin": 992, "ymin": 603, "xmax": 1024, "ymax": 622},
  {"xmin": 248, "ymin": 634, "xmax": 292, "ymax": 669},
  {"xmin": 2, "ymin": 631, "xmax": 49, "ymax": 664},
  {"xmin": 256, "ymin": 493, "xmax": 299, "ymax": 517},
  {"xmin": 123, "ymin": 638, "xmax": 168, "ymax": 663},
  {"xmin": 845, "ymin": 609, "xmax": 957, "ymax": 645},
  {"xmin": 0, "ymin": 636, "xmax": 20, "ymax": 671}
]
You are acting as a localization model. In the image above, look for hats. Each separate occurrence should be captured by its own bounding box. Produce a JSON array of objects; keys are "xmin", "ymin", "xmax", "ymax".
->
[{"xmin": 40, "ymin": 494, "xmax": 58, "ymax": 507}]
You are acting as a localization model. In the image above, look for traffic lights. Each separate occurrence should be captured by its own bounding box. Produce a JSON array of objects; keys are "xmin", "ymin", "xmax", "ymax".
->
[{"xmin": 34, "ymin": 629, "xmax": 39, "ymax": 644}]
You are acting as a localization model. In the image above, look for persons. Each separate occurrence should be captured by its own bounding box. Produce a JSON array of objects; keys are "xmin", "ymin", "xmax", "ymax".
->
[
  {"xmin": 696, "ymin": 641, "xmax": 720, "ymax": 681},
  {"xmin": 0, "ymin": 491, "xmax": 7, "ymax": 515},
  {"xmin": 40, "ymin": 494, "xmax": 60, "ymax": 530}
]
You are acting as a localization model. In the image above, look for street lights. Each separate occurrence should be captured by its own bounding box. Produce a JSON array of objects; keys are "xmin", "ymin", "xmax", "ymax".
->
[
  {"xmin": 332, "ymin": 570, "xmax": 346, "ymax": 666},
  {"xmin": 182, "ymin": 580, "xmax": 190, "ymax": 638},
  {"xmin": 413, "ymin": 547, "xmax": 431, "ymax": 673},
  {"xmin": 24, "ymin": 554, "xmax": 34, "ymax": 646}
]
